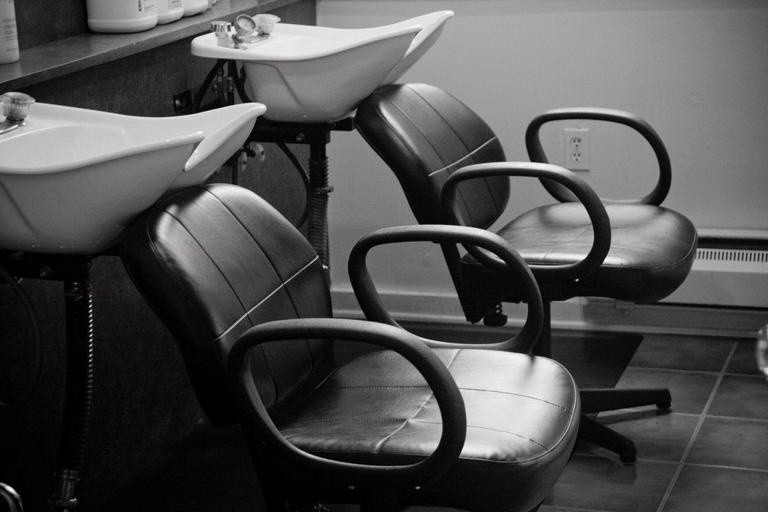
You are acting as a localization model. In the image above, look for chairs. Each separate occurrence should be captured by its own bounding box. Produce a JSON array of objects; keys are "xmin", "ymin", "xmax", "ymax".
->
[
  {"xmin": 354, "ymin": 81, "xmax": 697, "ymax": 466},
  {"xmin": 119, "ymin": 184, "xmax": 582, "ymax": 511}
]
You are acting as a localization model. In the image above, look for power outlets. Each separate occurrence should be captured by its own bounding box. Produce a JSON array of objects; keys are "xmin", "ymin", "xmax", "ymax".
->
[{"xmin": 563, "ymin": 129, "xmax": 591, "ymax": 170}]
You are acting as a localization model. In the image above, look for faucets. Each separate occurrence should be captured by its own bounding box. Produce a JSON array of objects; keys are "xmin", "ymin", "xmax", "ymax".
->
[{"xmin": 211, "ymin": 12, "xmax": 281, "ymax": 49}]
